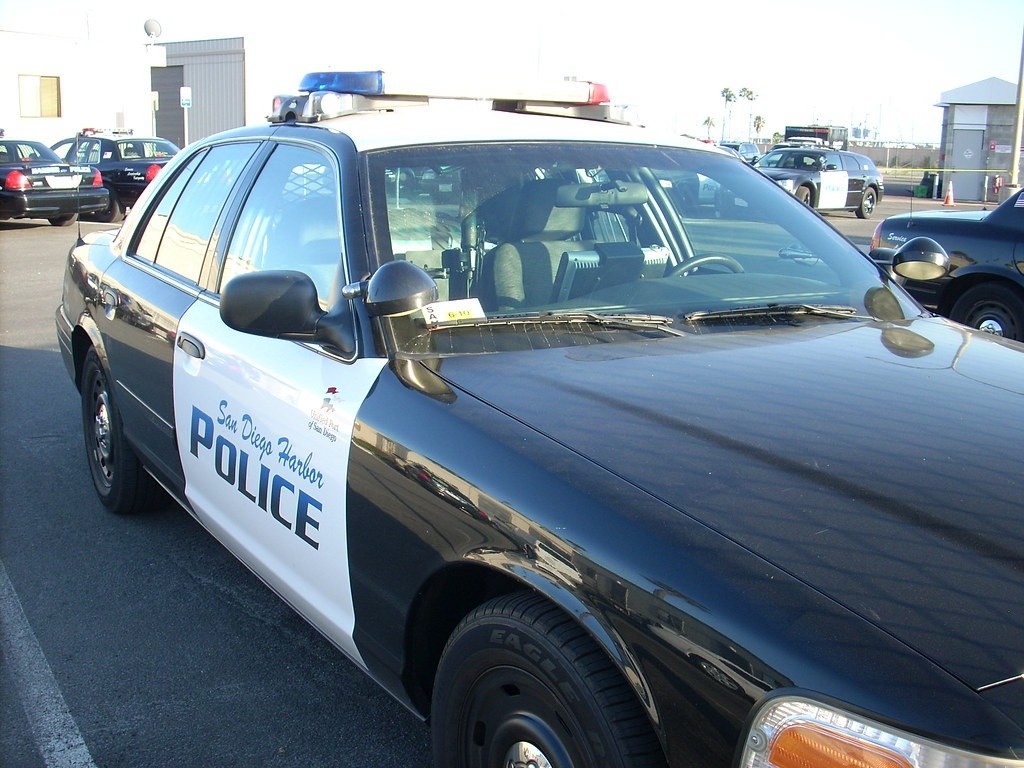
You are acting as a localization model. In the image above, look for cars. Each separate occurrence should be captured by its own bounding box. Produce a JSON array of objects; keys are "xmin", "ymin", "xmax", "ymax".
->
[
  {"xmin": 56, "ymin": 108, "xmax": 1024, "ymax": 768},
  {"xmin": 0, "ymin": 128, "xmax": 109, "ymax": 226},
  {"xmin": 750, "ymin": 143, "xmax": 834, "ymax": 168},
  {"xmin": 591, "ymin": 140, "xmax": 746, "ymax": 205},
  {"xmin": 713, "ymin": 147, "xmax": 884, "ymax": 223},
  {"xmin": 28, "ymin": 127, "xmax": 181, "ymax": 223},
  {"xmin": 422, "ymin": 158, "xmax": 596, "ymax": 209},
  {"xmin": 870, "ymin": 187, "xmax": 1024, "ymax": 343},
  {"xmin": 720, "ymin": 141, "xmax": 760, "ymax": 163}
]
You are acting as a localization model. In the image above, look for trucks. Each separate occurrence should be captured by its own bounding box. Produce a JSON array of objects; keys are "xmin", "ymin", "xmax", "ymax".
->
[{"xmin": 785, "ymin": 125, "xmax": 849, "ymax": 151}]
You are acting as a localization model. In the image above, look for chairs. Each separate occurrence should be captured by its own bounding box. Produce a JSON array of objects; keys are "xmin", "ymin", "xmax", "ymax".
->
[{"xmin": 492, "ymin": 178, "xmax": 602, "ymax": 312}]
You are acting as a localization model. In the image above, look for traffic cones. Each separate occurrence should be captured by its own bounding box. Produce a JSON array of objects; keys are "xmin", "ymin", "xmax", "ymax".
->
[{"xmin": 941, "ymin": 181, "xmax": 957, "ymax": 207}]
